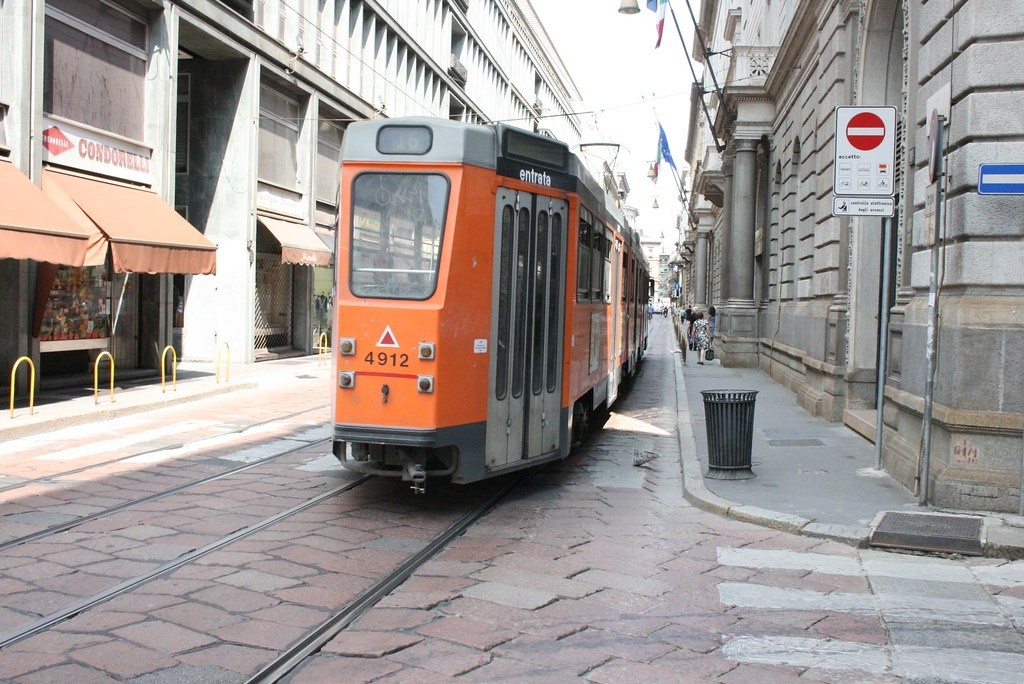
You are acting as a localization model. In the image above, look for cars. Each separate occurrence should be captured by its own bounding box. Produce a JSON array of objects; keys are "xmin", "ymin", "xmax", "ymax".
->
[{"xmin": 649, "ymin": 304, "xmax": 670, "ymax": 321}]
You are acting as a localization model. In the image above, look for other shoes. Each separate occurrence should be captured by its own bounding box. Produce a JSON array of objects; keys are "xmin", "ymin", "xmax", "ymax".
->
[{"xmin": 697, "ymin": 362, "xmax": 704, "ymax": 365}]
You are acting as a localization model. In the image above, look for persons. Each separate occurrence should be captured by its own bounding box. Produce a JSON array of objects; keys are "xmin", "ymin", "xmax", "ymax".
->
[
  {"xmin": 688, "ymin": 307, "xmax": 698, "ymax": 351},
  {"xmin": 664, "ymin": 307, "xmax": 667, "ymax": 315},
  {"xmin": 314, "ymin": 291, "xmax": 328, "ymax": 322},
  {"xmin": 681, "ymin": 307, "xmax": 685, "ymax": 324},
  {"xmin": 649, "ymin": 303, "xmax": 653, "ymax": 319},
  {"xmin": 686, "ymin": 305, "xmax": 692, "ymax": 316},
  {"xmin": 691, "ymin": 311, "xmax": 711, "ymax": 365},
  {"xmin": 707, "ymin": 306, "xmax": 715, "ymax": 346}
]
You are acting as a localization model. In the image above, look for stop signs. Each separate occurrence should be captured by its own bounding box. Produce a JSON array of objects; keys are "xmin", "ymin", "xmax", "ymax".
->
[{"xmin": 845, "ymin": 112, "xmax": 886, "ymax": 152}]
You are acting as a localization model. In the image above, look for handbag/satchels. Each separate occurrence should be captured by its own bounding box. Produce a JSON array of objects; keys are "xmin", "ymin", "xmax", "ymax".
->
[{"xmin": 705, "ymin": 340, "xmax": 714, "ymax": 360}]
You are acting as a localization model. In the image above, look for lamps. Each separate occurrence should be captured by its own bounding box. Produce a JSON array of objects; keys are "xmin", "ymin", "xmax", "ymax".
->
[
  {"xmin": 285, "ymin": 45, "xmax": 305, "ymax": 75},
  {"xmin": 373, "ymin": 104, "xmax": 386, "ymax": 119}
]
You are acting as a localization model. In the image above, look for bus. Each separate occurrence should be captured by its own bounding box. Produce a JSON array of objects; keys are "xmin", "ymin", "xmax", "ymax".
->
[{"xmin": 327, "ymin": 115, "xmax": 655, "ymax": 496}]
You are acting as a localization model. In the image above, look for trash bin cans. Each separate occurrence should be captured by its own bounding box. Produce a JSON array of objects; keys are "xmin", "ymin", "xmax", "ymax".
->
[{"xmin": 700, "ymin": 388, "xmax": 760, "ymax": 480}]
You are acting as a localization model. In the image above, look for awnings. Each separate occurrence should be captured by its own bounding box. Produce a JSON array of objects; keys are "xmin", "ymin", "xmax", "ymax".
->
[
  {"xmin": 0, "ymin": 161, "xmax": 90, "ymax": 267},
  {"xmin": 41, "ymin": 167, "xmax": 218, "ymax": 276},
  {"xmin": 258, "ymin": 215, "xmax": 332, "ymax": 267}
]
